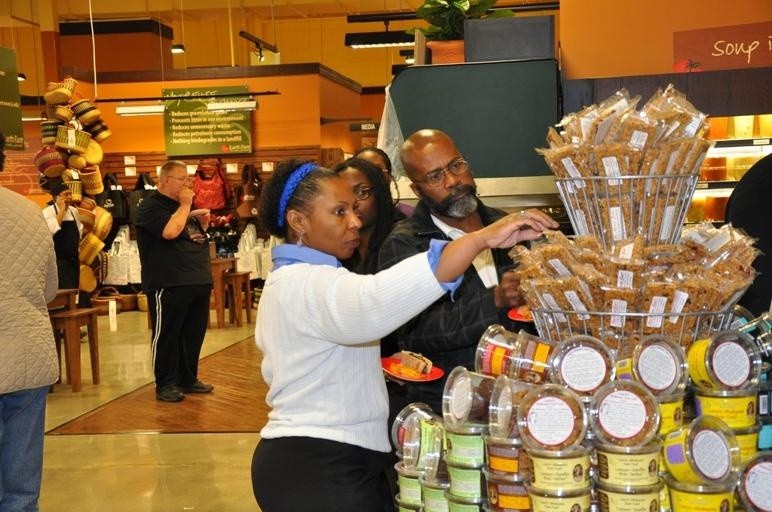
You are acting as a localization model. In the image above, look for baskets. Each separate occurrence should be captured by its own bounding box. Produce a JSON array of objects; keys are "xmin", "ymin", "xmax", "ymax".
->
[
  {"xmin": 81, "ymin": 138, "xmax": 103, "ymax": 165},
  {"xmin": 69, "ymin": 155, "xmax": 86, "ymax": 169},
  {"xmin": 79, "ymin": 165, "xmax": 104, "ymax": 195},
  {"xmin": 33, "ymin": 147, "xmax": 67, "ymax": 177},
  {"xmin": 79, "ymin": 265, "xmax": 96, "ymax": 292},
  {"xmin": 64, "ymin": 181, "xmax": 82, "ymax": 203},
  {"xmin": 138, "ymin": 291, "xmax": 149, "ymax": 311},
  {"xmin": 61, "ymin": 168, "xmax": 80, "ymax": 182},
  {"xmin": 77, "ymin": 196, "xmax": 95, "ymax": 211},
  {"xmin": 44, "ymin": 76, "xmax": 77, "ymax": 104},
  {"xmin": 79, "ymin": 233, "xmax": 105, "ymax": 266},
  {"xmin": 41, "ymin": 121, "xmax": 64, "ymax": 145},
  {"xmin": 55, "ymin": 121, "xmax": 91, "ymax": 154},
  {"xmin": 76, "ymin": 207, "xmax": 95, "ymax": 232},
  {"xmin": 120, "ymin": 287, "xmax": 139, "ymax": 311},
  {"xmin": 56, "ymin": 105, "xmax": 74, "ymax": 121},
  {"xmin": 92, "ymin": 206, "xmax": 112, "ymax": 240},
  {"xmin": 84, "ymin": 119, "xmax": 111, "ymax": 143},
  {"xmin": 70, "ymin": 99, "xmax": 102, "ymax": 125},
  {"xmin": 90, "ymin": 286, "xmax": 122, "ymax": 315}
]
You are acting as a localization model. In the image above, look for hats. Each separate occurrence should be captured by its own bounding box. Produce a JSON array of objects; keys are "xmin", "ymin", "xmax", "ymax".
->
[{"xmin": 51, "ymin": 183, "xmax": 68, "ymax": 194}]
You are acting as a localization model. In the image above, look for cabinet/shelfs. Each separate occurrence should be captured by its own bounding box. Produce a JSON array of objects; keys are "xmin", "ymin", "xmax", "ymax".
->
[{"xmin": 562, "ymin": 67, "xmax": 772, "ymax": 236}]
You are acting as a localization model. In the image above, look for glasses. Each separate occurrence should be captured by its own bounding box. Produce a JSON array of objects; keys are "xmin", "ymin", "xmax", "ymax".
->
[
  {"xmin": 356, "ymin": 186, "xmax": 372, "ymax": 200},
  {"xmin": 412, "ymin": 158, "xmax": 468, "ymax": 187},
  {"xmin": 167, "ymin": 175, "xmax": 192, "ymax": 183}
]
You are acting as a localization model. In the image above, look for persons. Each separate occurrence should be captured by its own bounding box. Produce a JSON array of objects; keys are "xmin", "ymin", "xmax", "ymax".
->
[
  {"xmin": 723, "ymin": 154, "xmax": 771, "ymax": 317},
  {"xmin": 42, "ymin": 184, "xmax": 86, "ymax": 342},
  {"xmin": 0, "ymin": 134, "xmax": 59, "ymax": 512},
  {"xmin": 378, "ymin": 130, "xmax": 532, "ymax": 414},
  {"xmin": 356, "ymin": 147, "xmax": 415, "ymax": 217},
  {"xmin": 136, "ymin": 160, "xmax": 215, "ymax": 403},
  {"xmin": 334, "ymin": 158, "xmax": 402, "ymax": 272},
  {"xmin": 252, "ymin": 159, "xmax": 559, "ymax": 512}
]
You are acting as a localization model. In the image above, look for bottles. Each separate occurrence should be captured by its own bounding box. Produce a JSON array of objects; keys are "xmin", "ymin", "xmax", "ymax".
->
[{"xmin": 209, "ymin": 239, "xmax": 216, "ymax": 260}]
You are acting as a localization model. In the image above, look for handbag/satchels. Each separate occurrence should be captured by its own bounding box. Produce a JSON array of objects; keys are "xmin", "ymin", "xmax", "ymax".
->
[
  {"xmin": 192, "ymin": 167, "xmax": 225, "ymax": 209},
  {"xmin": 196, "ymin": 159, "xmax": 233, "ymax": 199},
  {"xmin": 142, "ymin": 173, "xmax": 155, "ymax": 189},
  {"xmin": 235, "ymin": 164, "xmax": 263, "ymax": 218},
  {"xmin": 108, "ymin": 176, "xmax": 127, "ymax": 219},
  {"xmin": 96, "ymin": 175, "xmax": 124, "ymax": 217},
  {"xmin": 127, "ymin": 174, "xmax": 155, "ymax": 225}
]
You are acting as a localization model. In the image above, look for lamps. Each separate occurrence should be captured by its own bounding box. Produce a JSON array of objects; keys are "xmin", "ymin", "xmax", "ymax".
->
[
  {"xmin": 170, "ymin": 45, "xmax": 184, "ymax": 53},
  {"xmin": 208, "ymin": 102, "xmax": 257, "ymax": 112},
  {"xmin": 345, "ymin": 30, "xmax": 415, "ymax": 48},
  {"xmin": 18, "ymin": 74, "xmax": 27, "ymax": 81},
  {"xmin": 115, "ymin": 105, "xmax": 166, "ymax": 117},
  {"xmin": 254, "ymin": 44, "xmax": 265, "ymax": 62}
]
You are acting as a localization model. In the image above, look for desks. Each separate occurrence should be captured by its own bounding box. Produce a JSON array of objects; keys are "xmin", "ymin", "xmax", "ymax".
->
[{"xmin": 147, "ymin": 257, "xmax": 240, "ymax": 327}]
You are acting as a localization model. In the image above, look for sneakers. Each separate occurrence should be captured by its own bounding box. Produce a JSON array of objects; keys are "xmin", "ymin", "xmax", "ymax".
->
[
  {"xmin": 156, "ymin": 386, "xmax": 184, "ymax": 402},
  {"xmin": 174, "ymin": 379, "xmax": 213, "ymax": 393}
]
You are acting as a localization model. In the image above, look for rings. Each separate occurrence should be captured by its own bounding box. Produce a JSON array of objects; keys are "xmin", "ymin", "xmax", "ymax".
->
[{"xmin": 521, "ymin": 211, "xmax": 524, "ymax": 216}]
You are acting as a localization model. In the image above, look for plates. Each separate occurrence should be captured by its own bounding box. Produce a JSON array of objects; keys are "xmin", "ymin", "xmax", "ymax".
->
[{"xmin": 380, "ymin": 358, "xmax": 446, "ymax": 384}]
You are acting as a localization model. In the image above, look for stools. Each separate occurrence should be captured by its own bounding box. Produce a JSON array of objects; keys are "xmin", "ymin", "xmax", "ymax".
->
[
  {"xmin": 222, "ymin": 271, "xmax": 252, "ymax": 327},
  {"xmin": 49, "ymin": 308, "xmax": 101, "ymax": 393}
]
